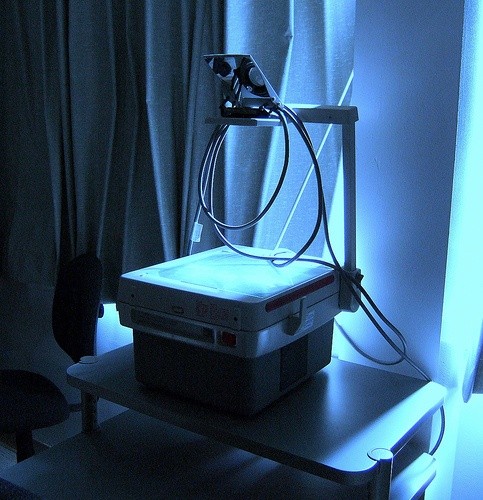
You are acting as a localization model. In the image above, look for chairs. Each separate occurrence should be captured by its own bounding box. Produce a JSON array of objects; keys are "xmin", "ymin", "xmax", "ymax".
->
[{"xmin": 0, "ymin": 253, "xmax": 103, "ymax": 462}]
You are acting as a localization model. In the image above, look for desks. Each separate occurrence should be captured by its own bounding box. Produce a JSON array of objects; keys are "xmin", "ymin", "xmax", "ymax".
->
[
  {"xmin": 66, "ymin": 343, "xmax": 447, "ymax": 500},
  {"xmin": 0, "ymin": 408, "xmax": 437, "ymax": 500}
]
